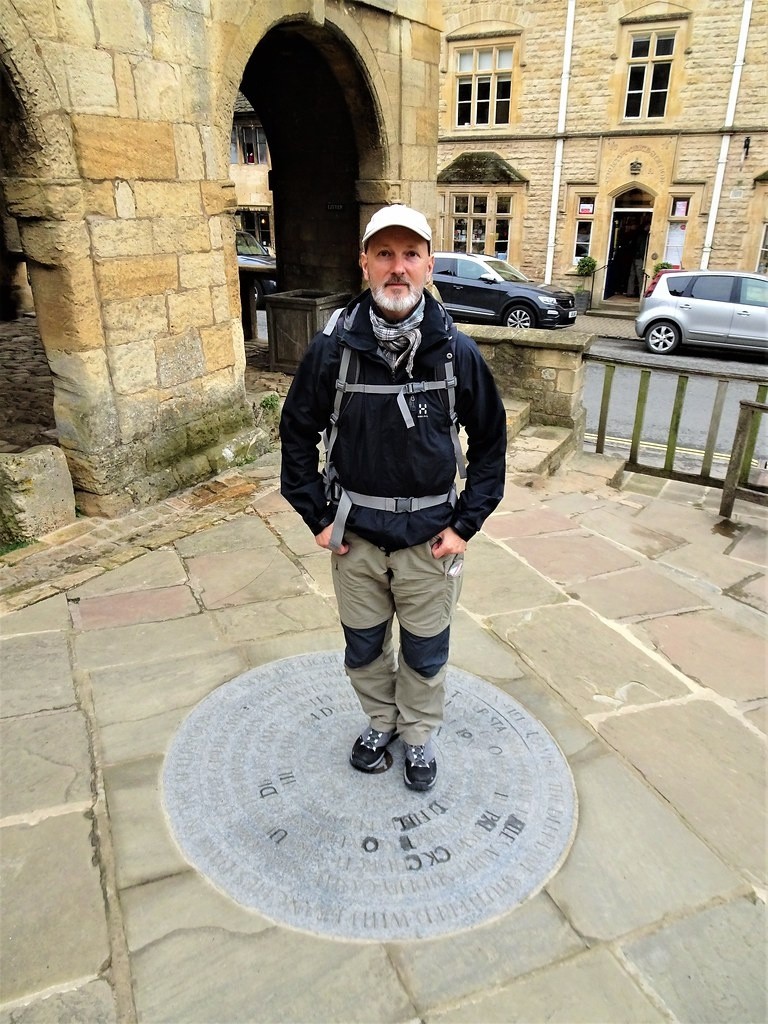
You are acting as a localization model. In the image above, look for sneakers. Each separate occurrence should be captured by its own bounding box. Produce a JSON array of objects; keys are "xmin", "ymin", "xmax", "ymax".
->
[
  {"xmin": 402, "ymin": 735, "xmax": 438, "ymax": 792},
  {"xmin": 350, "ymin": 722, "xmax": 398, "ymax": 772}
]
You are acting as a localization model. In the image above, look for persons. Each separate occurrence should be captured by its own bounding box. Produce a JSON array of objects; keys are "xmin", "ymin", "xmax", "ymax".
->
[
  {"xmin": 612, "ymin": 217, "xmax": 651, "ymax": 298},
  {"xmin": 280, "ymin": 203, "xmax": 507, "ymax": 790},
  {"xmin": 248, "ymin": 153, "xmax": 254, "ymax": 163}
]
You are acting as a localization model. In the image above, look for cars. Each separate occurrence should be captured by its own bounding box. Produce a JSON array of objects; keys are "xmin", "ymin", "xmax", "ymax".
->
[{"xmin": 633, "ymin": 270, "xmax": 768, "ymax": 357}]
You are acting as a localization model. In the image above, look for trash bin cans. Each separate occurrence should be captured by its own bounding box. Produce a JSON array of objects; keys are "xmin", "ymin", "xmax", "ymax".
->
[{"xmin": 263, "ymin": 288, "xmax": 351, "ymax": 376}]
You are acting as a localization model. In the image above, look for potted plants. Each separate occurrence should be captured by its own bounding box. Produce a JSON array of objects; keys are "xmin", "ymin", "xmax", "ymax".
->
[{"xmin": 573, "ymin": 256, "xmax": 597, "ymax": 316}]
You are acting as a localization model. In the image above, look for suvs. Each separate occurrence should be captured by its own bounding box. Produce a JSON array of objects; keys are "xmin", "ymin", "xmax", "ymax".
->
[
  {"xmin": 433, "ymin": 252, "xmax": 579, "ymax": 331},
  {"xmin": 234, "ymin": 230, "xmax": 278, "ymax": 310}
]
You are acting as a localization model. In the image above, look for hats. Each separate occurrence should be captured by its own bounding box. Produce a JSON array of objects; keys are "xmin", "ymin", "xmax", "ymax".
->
[{"xmin": 361, "ymin": 204, "xmax": 431, "ymax": 254}]
